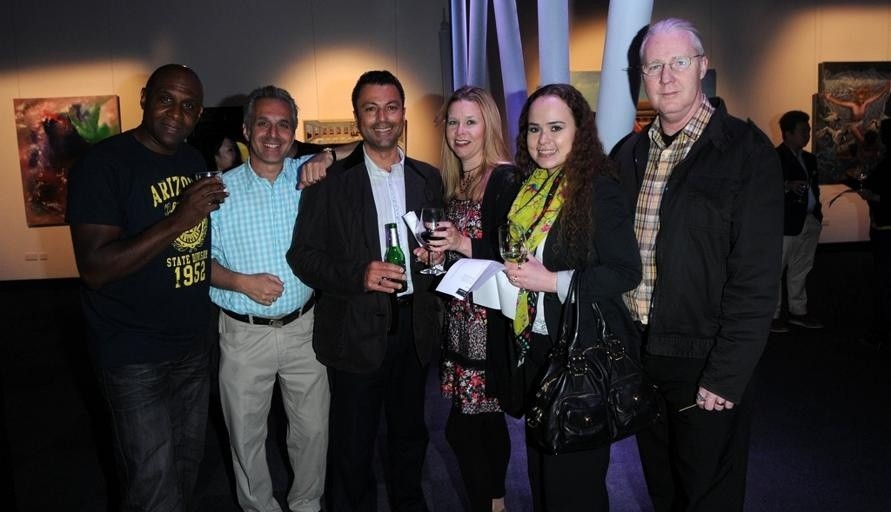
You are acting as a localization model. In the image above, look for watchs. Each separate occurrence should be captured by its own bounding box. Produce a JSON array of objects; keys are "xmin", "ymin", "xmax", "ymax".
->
[{"xmin": 323, "ymin": 147, "xmax": 337, "ymax": 164}]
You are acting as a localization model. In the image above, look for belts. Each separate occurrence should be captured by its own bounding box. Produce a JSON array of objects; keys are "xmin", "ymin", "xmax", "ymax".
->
[{"xmin": 222, "ymin": 291, "xmax": 315, "ymax": 328}]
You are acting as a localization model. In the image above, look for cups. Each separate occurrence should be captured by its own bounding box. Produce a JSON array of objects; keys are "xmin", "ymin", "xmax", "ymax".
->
[
  {"xmin": 194, "ymin": 170, "xmax": 224, "ymax": 206},
  {"xmin": 498, "ymin": 223, "xmax": 528, "ymax": 266}
]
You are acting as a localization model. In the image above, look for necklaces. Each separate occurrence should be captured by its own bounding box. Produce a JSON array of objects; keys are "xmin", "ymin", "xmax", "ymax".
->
[{"xmin": 459, "ymin": 164, "xmax": 485, "ymax": 193}]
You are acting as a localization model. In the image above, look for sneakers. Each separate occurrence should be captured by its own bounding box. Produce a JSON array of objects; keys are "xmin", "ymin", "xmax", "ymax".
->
[{"xmin": 771, "ymin": 318, "xmax": 818, "ymax": 334}]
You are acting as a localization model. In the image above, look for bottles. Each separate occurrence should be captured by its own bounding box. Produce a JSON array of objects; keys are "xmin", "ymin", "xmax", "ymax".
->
[{"xmin": 385, "ymin": 223, "xmax": 406, "ymax": 293}]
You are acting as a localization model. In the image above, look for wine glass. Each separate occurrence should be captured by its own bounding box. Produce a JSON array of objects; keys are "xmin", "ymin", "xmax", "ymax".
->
[{"xmin": 416, "ymin": 206, "xmax": 445, "ymax": 274}]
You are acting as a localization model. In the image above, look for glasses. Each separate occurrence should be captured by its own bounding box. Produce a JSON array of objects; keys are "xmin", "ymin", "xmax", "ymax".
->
[{"xmin": 642, "ymin": 51, "xmax": 708, "ymax": 76}]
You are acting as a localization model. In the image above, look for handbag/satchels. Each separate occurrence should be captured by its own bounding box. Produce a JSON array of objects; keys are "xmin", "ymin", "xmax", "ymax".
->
[{"xmin": 527, "ymin": 267, "xmax": 663, "ymax": 457}]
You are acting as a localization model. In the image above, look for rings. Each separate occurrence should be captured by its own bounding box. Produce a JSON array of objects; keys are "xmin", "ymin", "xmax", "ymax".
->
[
  {"xmin": 517, "ymin": 263, "xmax": 523, "ymax": 270},
  {"xmin": 696, "ymin": 392, "xmax": 705, "ymax": 403},
  {"xmin": 514, "ymin": 275, "xmax": 519, "ymax": 281},
  {"xmin": 715, "ymin": 400, "xmax": 726, "ymax": 406},
  {"xmin": 272, "ymin": 297, "xmax": 279, "ymax": 302},
  {"xmin": 379, "ymin": 277, "xmax": 387, "ymax": 286}
]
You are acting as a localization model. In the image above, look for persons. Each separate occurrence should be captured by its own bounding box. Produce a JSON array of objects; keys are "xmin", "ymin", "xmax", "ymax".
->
[
  {"xmin": 218, "ymin": 133, "xmax": 242, "ymax": 173},
  {"xmin": 416, "ymin": 85, "xmax": 526, "ymax": 510},
  {"xmin": 846, "ymin": 119, "xmax": 891, "ymax": 239},
  {"xmin": 289, "ymin": 71, "xmax": 446, "ymax": 510},
  {"xmin": 614, "ymin": 17, "xmax": 784, "ymax": 509},
  {"xmin": 68, "ymin": 64, "xmax": 228, "ymax": 511},
  {"xmin": 209, "ymin": 87, "xmax": 364, "ymax": 510},
  {"xmin": 503, "ymin": 85, "xmax": 641, "ymax": 511},
  {"xmin": 775, "ymin": 111, "xmax": 824, "ymax": 330}
]
